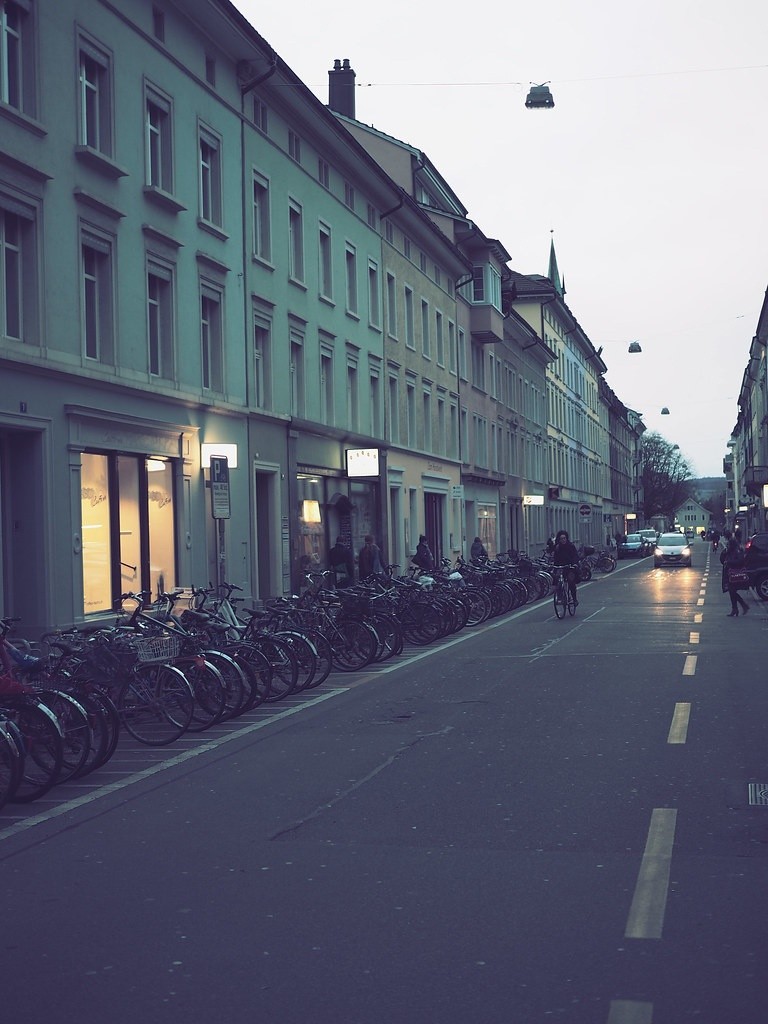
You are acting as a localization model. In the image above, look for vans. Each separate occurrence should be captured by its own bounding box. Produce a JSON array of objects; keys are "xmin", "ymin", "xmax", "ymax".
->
[
  {"xmin": 686, "ymin": 530, "xmax": 694, "ymax": 538},
  {"xmin": 635, "ymin": 529, "xmax": 657, "ymax": 550}
]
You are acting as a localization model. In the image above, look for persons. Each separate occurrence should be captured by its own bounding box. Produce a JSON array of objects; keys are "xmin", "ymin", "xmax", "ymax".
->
[
  {"xmin": 471, "ymin": 537, "xmax": 488, "ymax": 559},
  {"xmin": 547, "ymin": 533, "xmax": 556, "ymax": 555},
  {"xmin": 720, "ymin": 530, "xmax": 750, "ymax": 617},
  {"xmin": 417, "ymin": 535, "xmax": 435, "ymax": 571},
  {"xmin": 615, "ymin": 531, "xmax": 621, "ymax": 543},
  {"xmin": 329, "ymin": 536, "xmax": 354, "ymax": 575},
  {"xmin": 701, "ymin": 527, "xmax": 759, "ymax": 552},
  {"xmin": 554, "ymin": 530, "xmax": 579, "ymax": 607},
  {"xmin": 359, "ymin": 535, "xmax": 386, "ymax": 579}
]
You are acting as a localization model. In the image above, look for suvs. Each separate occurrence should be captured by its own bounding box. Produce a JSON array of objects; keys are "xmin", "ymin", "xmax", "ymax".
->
[
  {"xmin": 742, "ymin": 531, "xmax": 768, "ymax": 600},
  {"xmin": 653, "ymin": 533, "xmax": 694, "ymax": 567}
]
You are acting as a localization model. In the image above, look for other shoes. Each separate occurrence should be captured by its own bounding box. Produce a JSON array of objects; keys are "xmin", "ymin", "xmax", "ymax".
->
[{"xmin": 573, "ymin": 599, "xmax": 579, "ymax": 607}]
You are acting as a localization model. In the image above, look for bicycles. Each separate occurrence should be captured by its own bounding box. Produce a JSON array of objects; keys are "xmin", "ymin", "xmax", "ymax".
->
[
  {"xmin": 547, "ymin": 564, "xmax": 576, "ymax": 619},
  {"xmin": 399, "ymin": 551, "xmax": 554, "ymax": 646},
  {"xmin": 714, "ymin": 541, "xmax": 718, "ymax": 554},
  {"xmin": 88, "ymin": 566, "xmax": 404, "ymax": 746},
  {"xmin": 0, "ymin": 615, "xmax": 120, "ymax": 808},
  {"xmin": 578, "ymin": 544, "xmax": 617, "ymax": 581}
]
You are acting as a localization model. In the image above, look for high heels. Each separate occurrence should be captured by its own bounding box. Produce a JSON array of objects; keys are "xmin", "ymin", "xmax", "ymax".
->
[
  {"xmin": 742, "ymin": 604, "xmax": 750, "ymax": 615},
  {"xmin": 727, "ymin": 607, "xmax": 738, "ymax": 617}
]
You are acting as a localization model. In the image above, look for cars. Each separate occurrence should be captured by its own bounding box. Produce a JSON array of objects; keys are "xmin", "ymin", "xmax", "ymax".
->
[{"xmin": 620, "ymin": 534, "xmax": 650, "ymax": 558}]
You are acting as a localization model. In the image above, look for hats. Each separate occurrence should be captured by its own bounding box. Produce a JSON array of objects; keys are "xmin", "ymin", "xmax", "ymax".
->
[{"xmin": 419, "ymin": 534, "xmax": 427, "ymax": 543}]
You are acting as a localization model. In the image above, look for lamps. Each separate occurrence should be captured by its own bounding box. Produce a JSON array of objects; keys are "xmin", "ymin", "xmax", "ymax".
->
[
  {"xmin": 673, "ymin": 444, "xmax": 680, "ymax": 449},
  {"xmin": 682, "ymin": 464, "xmax": 687, "ymax": 467},
  {"xmin": 686, "ymin": 472, "xmax": 691, "ymax": 476},
  {"xmin": 147, "ymin": 460, "xmax": 165, "ymax": 472},
  {"xmin": 661, "ymin": 408, "xmax": 670, "ymax": 414},
  {"xmin": 629, "ymin": 343, "xmax": 642, "ymax": 353}
]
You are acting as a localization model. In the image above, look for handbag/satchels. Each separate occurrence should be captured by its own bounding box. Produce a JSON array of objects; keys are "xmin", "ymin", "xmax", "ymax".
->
[{"xmin": 728, "ymin": 567, "xmax": 749, "ymax": 585}]
